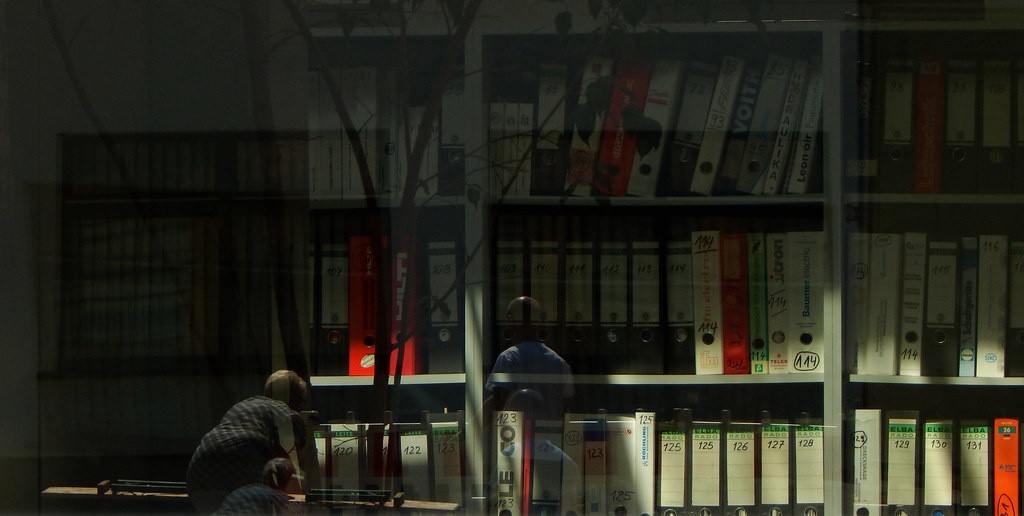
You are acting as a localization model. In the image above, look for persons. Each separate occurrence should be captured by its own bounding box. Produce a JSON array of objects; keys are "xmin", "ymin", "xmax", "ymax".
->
[
  {"xmin": 186, "ymin": 369, "xmax": 321, "ymax": 516},
  {"xmin": 446, "ymin": 296, "xmax": 581, "ymax": 516}
]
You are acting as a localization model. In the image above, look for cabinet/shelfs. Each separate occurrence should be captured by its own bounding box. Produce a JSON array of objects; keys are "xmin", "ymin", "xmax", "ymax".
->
[{"xmin": 304, "ymin": 18, "xmax": 1024, "ymax": 516}]
